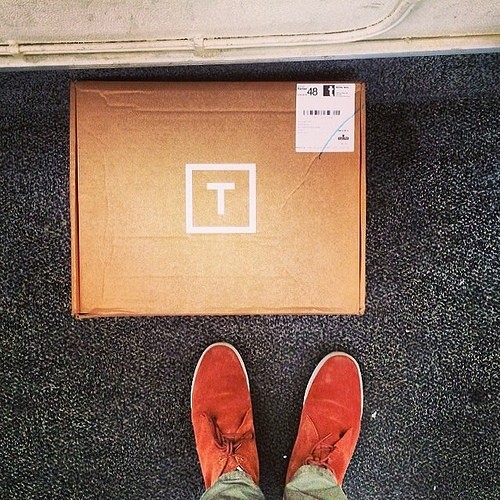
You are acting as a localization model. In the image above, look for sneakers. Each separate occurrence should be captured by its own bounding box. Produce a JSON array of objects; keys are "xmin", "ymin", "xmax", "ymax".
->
[
  {"xmin": 190, "ymin": 343, "xmax": 261, "ymax": 488},
  {"xmin": 284, "ymin": 350, "xmax": 364, "ymax": 488}
]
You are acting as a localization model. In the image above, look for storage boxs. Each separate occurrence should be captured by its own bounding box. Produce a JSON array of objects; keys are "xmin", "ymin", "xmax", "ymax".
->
[{"xmin": 69, "ymin": 81, "xmax": 367, "ymax": 319}]
